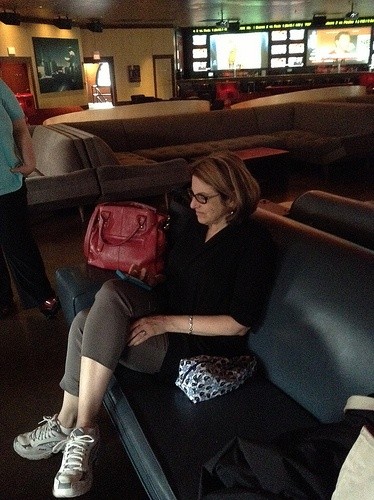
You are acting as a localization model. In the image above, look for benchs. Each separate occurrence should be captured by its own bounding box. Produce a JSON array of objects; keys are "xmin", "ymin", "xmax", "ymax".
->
[{"xmin": 25, "ymin": 85, "xmax": 374, "ymax": 500}]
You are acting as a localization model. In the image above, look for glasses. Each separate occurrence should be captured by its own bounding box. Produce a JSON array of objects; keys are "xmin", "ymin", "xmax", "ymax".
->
[{"xmin": 187, "ymin": 187, "xmax": 219, "ymax": 204}]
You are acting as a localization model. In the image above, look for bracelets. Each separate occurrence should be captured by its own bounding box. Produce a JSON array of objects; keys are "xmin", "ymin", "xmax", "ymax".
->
[{"xmin": 189, "ymin": 316, "xmax": 193, "ymax": 335}]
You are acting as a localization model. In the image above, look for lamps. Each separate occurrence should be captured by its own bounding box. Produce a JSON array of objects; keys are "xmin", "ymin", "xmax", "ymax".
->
[
  {"xmin": 55, "ymin": 15, "xmax": 73, "ymax": 29},
  {"xmin": 309, "ymin": 12, "xmax": 327, "ymax": 29},
  {"xmin": 86, "ymin": 18, "xmax": 102, "ymax": 32},
  {"xmin": 0, "ymin": 8, "xmax": 20, "ymax": 26},
  {"xmin": 226, "ymin": 17, "xmax": 240, "ymax": 32},
  {"xmin": 92, "ymin": 32, "xmax": 100, "ymax": 60}
]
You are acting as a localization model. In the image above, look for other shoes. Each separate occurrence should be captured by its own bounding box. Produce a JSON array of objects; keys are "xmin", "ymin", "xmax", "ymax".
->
[
  {"xmin": 0, "ymin": 295, "xmax": 14, "ymax": 316},
  {"xmin": 38, "ymin": 298, "xmax": 59, "ymax": 320}
]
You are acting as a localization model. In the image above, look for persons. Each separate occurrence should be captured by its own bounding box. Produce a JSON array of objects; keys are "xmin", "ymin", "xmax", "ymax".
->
[
  {"xmin": 0, "ymin": 79, "xmax": 56, "ymax": 320},
  {"xmin": 13, "ymin": 150, "xmax": 281, "ymax": 497},
  {"xmin": 321, "ymin": 32, "xmax": 355, "ymax": 62},
  {"xmin": 64, "ymin": 46, "xmax": 76, "ymax": 72}
]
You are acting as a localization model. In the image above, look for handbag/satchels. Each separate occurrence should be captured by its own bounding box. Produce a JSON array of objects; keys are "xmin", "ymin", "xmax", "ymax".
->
[
  {"xmin": 175, "ymin": 354, "xmax": 258, "ymax": 403},
  {"xmin": 83, "ymin": 200, "xmax": 170, "ymax": 276}
]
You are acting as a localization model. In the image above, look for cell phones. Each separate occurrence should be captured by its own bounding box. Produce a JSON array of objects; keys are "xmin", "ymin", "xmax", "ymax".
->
[{"xmin": 115, "ymin": 270, "xmax": 152, "ymax": 291}]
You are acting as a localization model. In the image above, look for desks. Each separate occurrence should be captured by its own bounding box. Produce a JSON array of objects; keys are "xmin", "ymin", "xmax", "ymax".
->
[{"xmin": 237, "ymin": 147, "xmax": 291, "ymax": 164}]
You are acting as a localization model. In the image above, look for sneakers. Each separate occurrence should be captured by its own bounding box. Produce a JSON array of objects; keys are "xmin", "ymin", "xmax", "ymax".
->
[
  {"xmin": 52, "ymin": 423, "xmax": 101, "ymax": 497},
  {"xmin": 12, "ymin": 415, "xmax": 76, "ymax": 460}
]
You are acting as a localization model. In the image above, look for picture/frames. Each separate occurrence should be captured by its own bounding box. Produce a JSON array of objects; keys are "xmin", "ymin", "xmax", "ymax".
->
[{"xmin": 31, "ymin": 36, "xmax": 87, "ymax": 96}]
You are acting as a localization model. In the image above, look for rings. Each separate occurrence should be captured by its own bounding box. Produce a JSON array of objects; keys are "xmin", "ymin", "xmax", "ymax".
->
[{"xmin": 143, "ymin": 330, "xmax": 146, "ymax": 335}]
